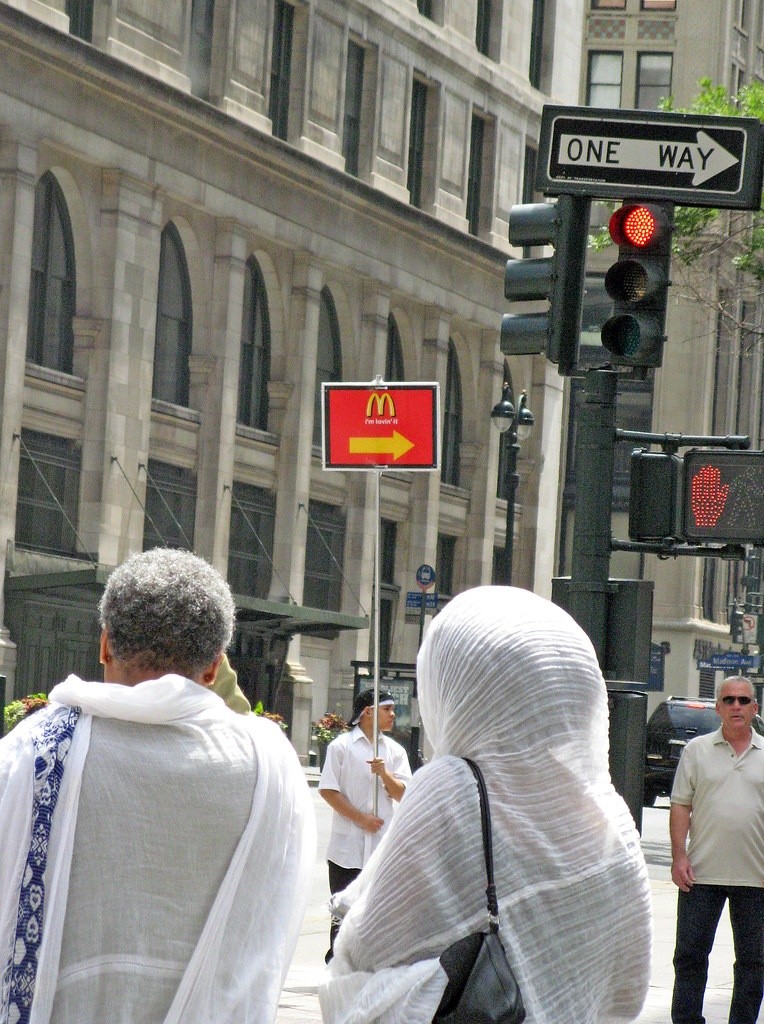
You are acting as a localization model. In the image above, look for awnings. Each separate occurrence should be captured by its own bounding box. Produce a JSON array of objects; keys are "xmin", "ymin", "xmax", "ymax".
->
[{"xmin": 5, "ymin": 568, "xmax": 370, "ymax": 640}]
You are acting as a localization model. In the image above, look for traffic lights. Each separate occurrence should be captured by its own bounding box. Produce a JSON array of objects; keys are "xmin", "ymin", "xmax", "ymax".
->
[
  {"xmin": 601, "ymin": 197, "xmax": 674, "ymax": 366},
  {"xmin": 500, "ymin": 194, "xmax": 592, "ymax": 363},
  {"xmin": 681, "ymin": 449, "xmax": 764, "ymax": 544}
]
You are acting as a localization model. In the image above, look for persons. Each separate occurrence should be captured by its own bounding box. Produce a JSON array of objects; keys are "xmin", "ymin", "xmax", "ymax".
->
[
  {"xmin": 318, "ymin": 585, "xmax": 651, "ymax": 1023},
  {"xmin": 668, "ymin": 676, "xmax": 764, "ymax": 1023},
  {"xmin": 0, "ymin": 547, "xmax": 312, "ymax": 1024},
  {"xmin": 319, "ymin": 687, "xmax": 412, "ymax": 964}
]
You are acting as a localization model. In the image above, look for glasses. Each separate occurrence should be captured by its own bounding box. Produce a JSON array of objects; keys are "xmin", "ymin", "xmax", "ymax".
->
[{"xmin": 723, "ymin": 696, "xmax": 751, "ymax": 705}]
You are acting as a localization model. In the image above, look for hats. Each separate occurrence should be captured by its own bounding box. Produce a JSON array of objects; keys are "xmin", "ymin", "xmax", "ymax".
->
[{"xmin": 347, "ymin": 688, "xmax": 394, "ymax": 727}]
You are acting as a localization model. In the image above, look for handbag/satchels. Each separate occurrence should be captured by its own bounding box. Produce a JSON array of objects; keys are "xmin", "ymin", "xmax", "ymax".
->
[{"xmin": 431, "ymin": 756, "xmax": 526, "ymax": 1024}]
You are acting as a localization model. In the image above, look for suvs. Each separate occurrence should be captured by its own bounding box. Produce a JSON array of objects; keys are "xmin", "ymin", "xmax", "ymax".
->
[{"xmin": 643, "ymin": 694, "xmax": 763, "ymax": 808}]
